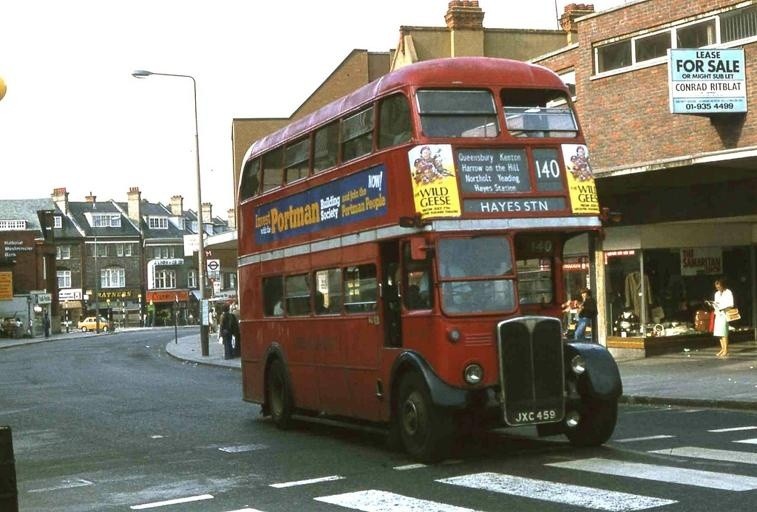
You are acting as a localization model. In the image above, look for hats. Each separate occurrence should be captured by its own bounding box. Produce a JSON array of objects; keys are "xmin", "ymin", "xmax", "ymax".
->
[{"xmin": 578, "ymin": 288, "xmax": 590, "ymax": 294}]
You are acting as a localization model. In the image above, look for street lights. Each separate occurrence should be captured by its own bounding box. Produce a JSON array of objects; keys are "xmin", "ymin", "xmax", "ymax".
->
[{"xmin": 132, "ymin": 69, "xmax": 209, "ymax": 357}]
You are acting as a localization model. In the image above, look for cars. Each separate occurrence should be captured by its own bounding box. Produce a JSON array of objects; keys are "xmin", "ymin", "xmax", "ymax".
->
[{"xmin": 78, "ymin": 316, "xmax": 109, "ymax": 332}]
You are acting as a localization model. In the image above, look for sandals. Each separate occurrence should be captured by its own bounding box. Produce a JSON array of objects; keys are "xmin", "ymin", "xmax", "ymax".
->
[{"xmin": 716, "ymin": 351, "xmax": 728, "ymax": 357}]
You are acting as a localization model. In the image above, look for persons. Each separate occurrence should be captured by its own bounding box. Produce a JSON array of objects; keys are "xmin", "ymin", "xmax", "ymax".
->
[
  {"xmin": 573, "ymin": 288, "xmax": 596, "ymax": 341},
  {"xmin": 413, "ymin": 146, "xmax": 448, "ymax": 185},
  {"xmin": 418, "ymin": 239, "xmax": 474, "ymax": 312},
  {"xmin": 209, "ymin": 299, "xmax": 241, "ymax": 361},
  {"xmin": 16, "ymin": 317, "xmax": 23, "ymax": 328},
  {"xmin": 711, "ymin": 278, "xmax": 734, "ymax": 357},
  {"xmin": 43, "ymin": 314, "xmax": 50, "ymax": 337},
  {"xmin": 569, "ymin": 146, "xmax": 593, "ymax": 182}
]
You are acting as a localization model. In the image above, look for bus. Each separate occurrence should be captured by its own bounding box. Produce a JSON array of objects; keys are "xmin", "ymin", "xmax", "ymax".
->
[{"xmin": 236, "ymin": 57, "xmax": 623, "ymax": 461}]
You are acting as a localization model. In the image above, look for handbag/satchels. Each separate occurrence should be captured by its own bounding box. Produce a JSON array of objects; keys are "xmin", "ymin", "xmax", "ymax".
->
[{"xmin": 724, "ymin": 308, "xmax": 740, "ymax": 323}]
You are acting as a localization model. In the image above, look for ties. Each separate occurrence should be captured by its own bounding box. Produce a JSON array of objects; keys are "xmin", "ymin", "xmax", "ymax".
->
[{"xmin": 442, "ymin": 266, "xmax": 452, "ymax": 307}]
